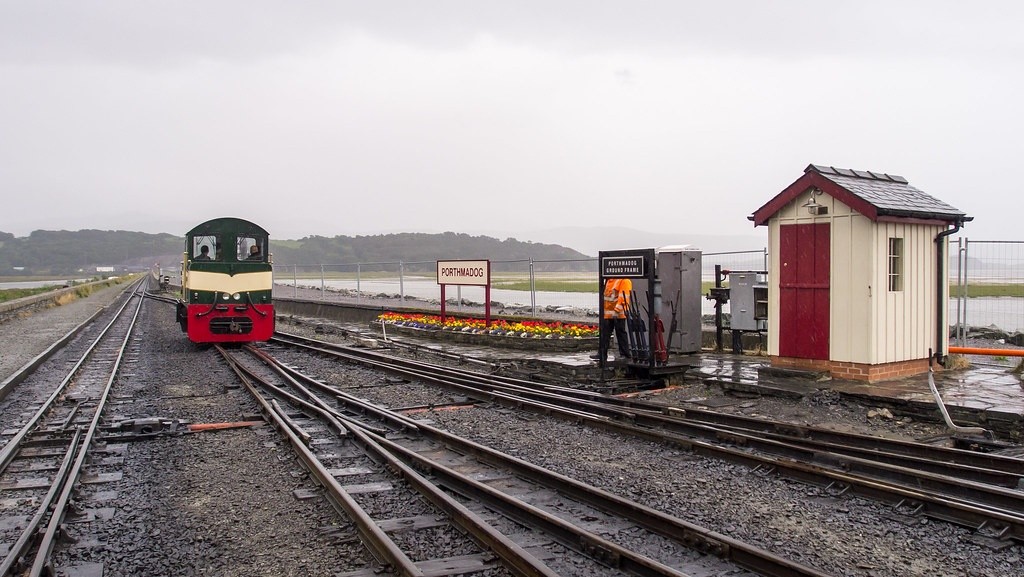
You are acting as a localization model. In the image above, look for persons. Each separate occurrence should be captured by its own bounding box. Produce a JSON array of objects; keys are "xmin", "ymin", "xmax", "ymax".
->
[
  {"xmin": 589, "ymin": 277, "xmax": 633, "ymax": 359},
  {"xmin": 196, "ymin": 246, "xmax": 211, "ymax": 261},
  {"xmin": 246, "ymin": 245, "xmax": 263, "ymax": 261}
]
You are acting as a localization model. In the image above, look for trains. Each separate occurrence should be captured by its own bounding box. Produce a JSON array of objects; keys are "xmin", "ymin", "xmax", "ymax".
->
[{"xmin": 173, "ymin": 215, "xmax": 276, "ymax": 350}]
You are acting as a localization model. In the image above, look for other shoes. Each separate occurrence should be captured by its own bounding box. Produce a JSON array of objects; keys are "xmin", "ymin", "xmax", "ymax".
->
[{"xmin": 590, "ymin": 354, "xmax": 607, "ymax": 360}]
